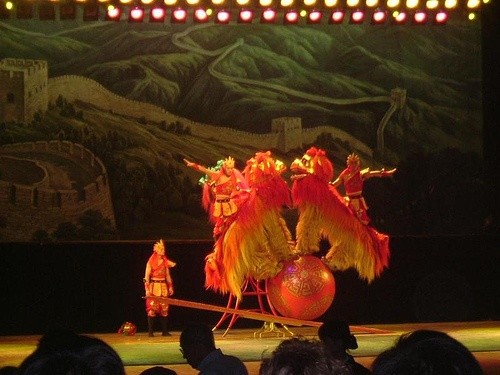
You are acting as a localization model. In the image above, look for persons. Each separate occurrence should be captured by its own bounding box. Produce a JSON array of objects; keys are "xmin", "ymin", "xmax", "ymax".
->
[
  {"xmin": 183, "ymin": 155, "xmax": 252, "ymax": 232},
  {"xmin": 144, "ymin": 240, "xmax": 176, "ymax": 336},
  {"xmin": 258, "ymin": 320, "xmax": 485, "ymax": 375},
  {"xmin": 330, "ymin": 152, "xmax": 397, "ymax": 224},
  {"xmin": 179, "ymin": 322, "xmax": 248, "ymax": 375},
  {"xmin": 0, "ymin": 329, "xmax": 177, "ymax": 375}
]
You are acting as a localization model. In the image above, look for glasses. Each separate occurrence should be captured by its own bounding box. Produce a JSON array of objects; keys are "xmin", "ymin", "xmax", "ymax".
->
[{"xmin": 179, "ymin": 349, "xmax": 186, "ymax": 356}]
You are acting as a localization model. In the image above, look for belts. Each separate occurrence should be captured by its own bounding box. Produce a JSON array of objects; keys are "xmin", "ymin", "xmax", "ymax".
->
[
  {"xmin": 151, "ymin": 280, "xmax": 166, "ymax": 283},
  {"xmin": 216, "ymin": 198, "xmax": 230, "ymax": 203},
  {"xmin": 348, "ymin": 194, "xmax": 363, "ymax": 199}
]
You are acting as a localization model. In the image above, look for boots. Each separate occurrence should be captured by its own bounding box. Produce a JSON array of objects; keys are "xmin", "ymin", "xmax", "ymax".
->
[
  {"xmin": 160, "ymin": 316, "xmax": 172, "ymax": 336},
  {"xmin": 147, "ymin": 316, "xmax": 156, "ymax": 337}
]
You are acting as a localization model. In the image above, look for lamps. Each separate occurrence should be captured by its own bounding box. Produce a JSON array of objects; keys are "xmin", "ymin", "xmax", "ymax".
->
[
  {"xmin": 17, "ymin": 0, "xmax": 33, "ymax": 19},
  {"xmin": 83, "ymin": 0, "xmax": 100, "ymax": 21},
  {"xmin": 39, "ymin": 0, "xmax": 56, "ymax": 20},
  {"xmin": 130, "ymin": 2, "xmax": 145, "ymax": 21},
  {"xmin": 60, "ymin": 0, "xmax": 78, "ymax": 19},
  {"xmin": 106, "ymin": 0, "xmax": 124, "ymax": 22}
]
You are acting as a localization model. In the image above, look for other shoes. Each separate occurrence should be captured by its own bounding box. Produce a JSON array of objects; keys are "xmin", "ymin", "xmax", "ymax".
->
[{"xmin": 320, "ymin": 256, "xmax": 338, "ymax": 272}]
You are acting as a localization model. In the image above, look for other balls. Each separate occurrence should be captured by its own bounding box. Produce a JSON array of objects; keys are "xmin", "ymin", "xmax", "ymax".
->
[{"xmin": 267, "ymin": 255, "xmax": 335, "ymax": 321}]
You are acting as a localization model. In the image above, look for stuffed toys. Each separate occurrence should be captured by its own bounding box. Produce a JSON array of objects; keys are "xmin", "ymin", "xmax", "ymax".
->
[
  {"xmin": 290, "ymin": 146, "xmax": 390, "ymax": 282},
  {"xmin": 205, "ymin": 151, "xmax": 296, "ymax": 298}
]
